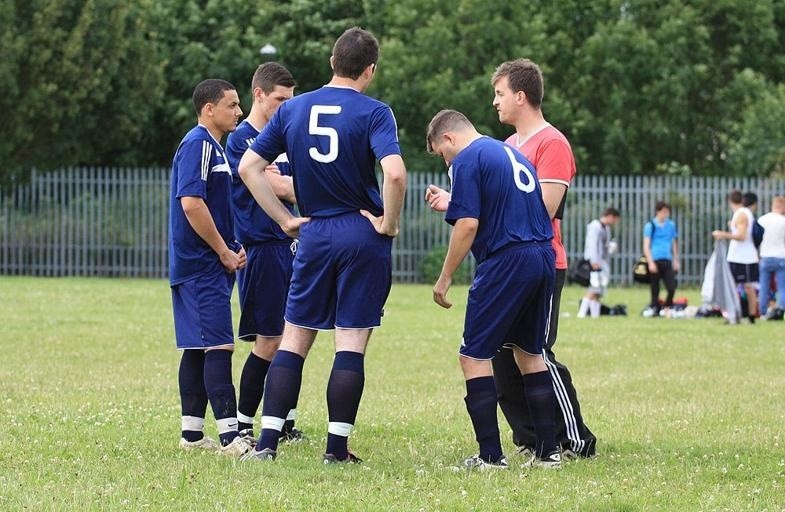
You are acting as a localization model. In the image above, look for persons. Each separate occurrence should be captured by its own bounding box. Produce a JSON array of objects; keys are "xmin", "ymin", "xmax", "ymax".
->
[
  {"xmin": 168, "ymin": 79, "xmax": 254, "ymax": 461},
  {"xmin": 226, "ymin": 62, "xmax": 307, "ymax": 451},
  {"xmin": 712, "ymin": 190, "xmax": 785, "ymax": 323},
  {"xmin": 425, "ymin": 59, "xmax": 596, "ymax": 458},
  {"xmin": 577, "ymin": 208, "xmax": 619, "ymax": 318},
  {"xmin": 425, "ymin": 109, "xmax": 562, "ymax": 471},
  {"xmin": 238, "ymin": 27, "xmax": 406, "ymax": 468},
  {"xmin": 643, "ymin": 203, "xmax": 679, "ymax": 316}
]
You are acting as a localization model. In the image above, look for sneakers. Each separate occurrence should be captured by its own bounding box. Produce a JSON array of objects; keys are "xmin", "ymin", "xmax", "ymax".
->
[
  {"xmin": 180, "ymin": 428, "xmax": 363, "ymax": 464},
  {"xmin": 456, "ymin": 444, "xmax": 595, "ymax": 470}
]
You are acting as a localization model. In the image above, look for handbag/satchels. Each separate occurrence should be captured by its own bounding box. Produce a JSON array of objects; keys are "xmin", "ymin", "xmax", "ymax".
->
[
  {"xmin": 569, "ymin": 261, "xmax": 591, "ymax": 286},
  {"xmin": 633, "ymin": 257, "xmax": 649, "ymax": 283}
]
